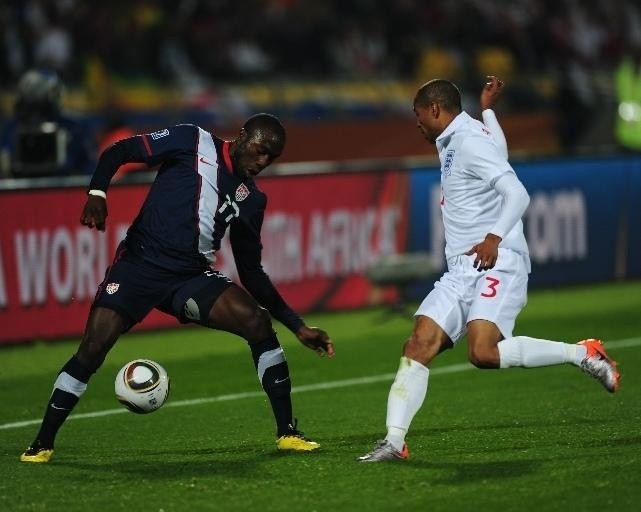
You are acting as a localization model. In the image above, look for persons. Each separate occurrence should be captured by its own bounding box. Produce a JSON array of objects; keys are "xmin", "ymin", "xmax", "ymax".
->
[
  {"xmin": 19, "ymin": 112, "xmax": 335, "ymax": 464},
  {"xmin": 359, "ymin": 73, "xmax": 621, "ymax": 462},
  {"xmin": 2, "ymin": 2, "xmax": 640, "ymax": 179}
]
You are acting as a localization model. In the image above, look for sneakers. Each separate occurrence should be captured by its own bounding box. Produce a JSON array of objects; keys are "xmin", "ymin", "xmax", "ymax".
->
[
  {"xmin": 576, "ymin": 338, "xmax": 620, "ymax": 393},
  {"xmin": 21, "ymin": 437, "xmax": 56, "ymax": 463},
  {"xmin": 275, "ymin": 417, "xmax": 320, "ymax": 452},
  {"xmin": 357, "ymin": 438, "xmax": 410, "ymax": 463}
]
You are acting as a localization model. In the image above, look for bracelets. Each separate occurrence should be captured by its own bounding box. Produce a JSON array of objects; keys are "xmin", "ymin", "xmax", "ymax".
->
[{"xmin": 88, "ymin": 189, "xmax": 106, "ymax": 199}]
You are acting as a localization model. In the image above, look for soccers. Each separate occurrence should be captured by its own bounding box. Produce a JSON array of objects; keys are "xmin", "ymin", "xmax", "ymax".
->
[{"xmin": 115, "ymin": 359, "xmax": 171, "ymax": 415}]
